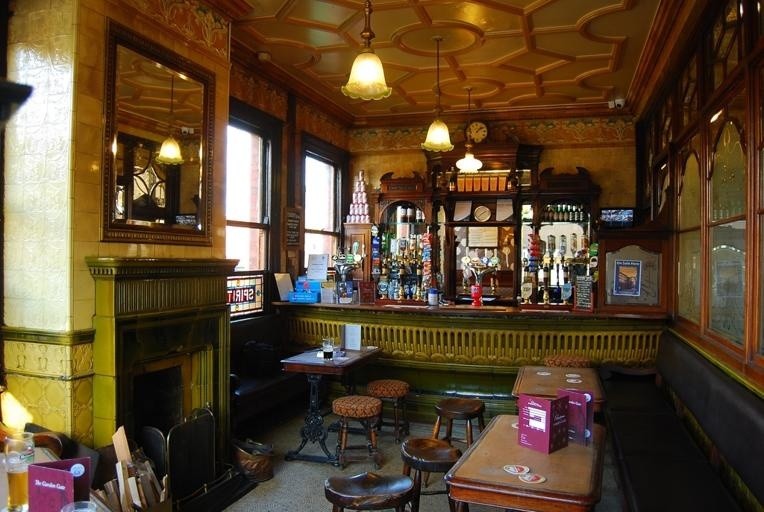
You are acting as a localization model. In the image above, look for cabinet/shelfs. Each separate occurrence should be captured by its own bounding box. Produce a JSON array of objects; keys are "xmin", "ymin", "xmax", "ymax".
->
[
  {"xmin": 342, "ymin": 221, "xmax": 372, "ymax": 282},
  {"xmin": 370, "ymin": 194, "xmax": 596, "ymax": 310}
]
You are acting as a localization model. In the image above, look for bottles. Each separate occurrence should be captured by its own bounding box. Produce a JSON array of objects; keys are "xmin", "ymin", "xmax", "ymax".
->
[
  {"xmin": 528, "ymin": 198, "xmax": 591, "ymax": 308},
  {"xmin": 507, "ymin": 168, "xmax": 520, "ymax": 193},
  {"xmin": 448, "ymin": 166, "xmax": 457, "ymax": 192},
  {"xmin": 371, "ymin": 201, "xmax": 440, "ymax": 308},
  {"xmin": 346, "ymin": 168, "xmax": 371, "ymax": 224}
]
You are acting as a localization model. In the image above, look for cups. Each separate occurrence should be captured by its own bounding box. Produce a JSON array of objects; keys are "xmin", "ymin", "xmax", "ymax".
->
[
  {"xmin": 59, "ymin": 501, "xmax": 97, "ymax": 512},
  {"xmin": 3, "ymin": 431, "xmax": 37, "ymax": 512},
  {"xmin": 322, "ymin": 335, "xmax": 335, "ymax": 362},
  {"xmin": 470, "ymin": 286, "xmax": 483, "ymax": 306}
]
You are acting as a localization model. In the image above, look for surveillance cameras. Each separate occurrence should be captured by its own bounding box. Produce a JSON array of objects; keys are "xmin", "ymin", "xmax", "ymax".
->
[{"xmin": 614, "ymin": 99, "xmax": 625, "ymax": 109}]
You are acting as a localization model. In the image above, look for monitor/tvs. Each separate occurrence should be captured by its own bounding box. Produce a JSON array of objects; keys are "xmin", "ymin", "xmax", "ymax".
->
[{"xmin": 599, "ymin": 207, "xmax": 636, "ymax": 228}]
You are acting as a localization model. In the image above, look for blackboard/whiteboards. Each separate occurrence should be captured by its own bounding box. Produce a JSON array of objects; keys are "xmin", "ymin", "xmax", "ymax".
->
[{"xmin": 576, "ymin": 275, "xmax": 592, "ymax": 308}]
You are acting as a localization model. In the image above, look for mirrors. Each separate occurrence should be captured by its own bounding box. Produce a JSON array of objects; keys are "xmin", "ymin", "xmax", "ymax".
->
[{"xmin": 100, "ymin": 15, "xmax": 217, "ymax": 247}]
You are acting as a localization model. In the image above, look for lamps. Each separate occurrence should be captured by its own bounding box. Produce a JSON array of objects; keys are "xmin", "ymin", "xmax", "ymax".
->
[
  {"xmin": 420, "ymin": 37, "xmax": 455, "ymax": 153},
  {"xmin": 154, "ymin": 74, "xmax": 186, "ymax": 166},
  {"xmin": 455, "ymin": 88, "xmax": 483, "ymax": 170},
  {"xmin": 341, "ymin": 0, "xmax": 393, "ymax": 102}
]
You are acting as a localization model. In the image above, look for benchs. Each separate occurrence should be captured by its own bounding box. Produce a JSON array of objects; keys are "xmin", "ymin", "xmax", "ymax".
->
[
  {"xmin": 599, "ymin": 331, "xmax": 764, "ymax": 512},
  {"xmin": 230, "ymin": 312, "xmax": 316, "ymax": 434}
]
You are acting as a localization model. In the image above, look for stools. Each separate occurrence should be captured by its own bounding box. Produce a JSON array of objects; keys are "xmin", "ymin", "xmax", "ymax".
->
[
  {"xmin": 399, "ymin": 436, "xmax": 470, "ymax": 512},
  {"xmin": 367, "ymin": 379, "xmax": 410, "ymax": 445},
  {"xmin": 323, "ymin": 470, "xmax": 415, "ymax": 511},
  {"xmin": 423, "ymin": 397, "xmax": 485, "ymax": 488},
  {"xmin": 331, "ymin": 395, "xmax": 383, "ymax": 471}
]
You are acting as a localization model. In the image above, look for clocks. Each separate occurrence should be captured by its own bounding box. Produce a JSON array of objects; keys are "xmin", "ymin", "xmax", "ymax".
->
[{"xmin": 464, "ymin": 120, "xmax": 489, "ymax": 145}]
